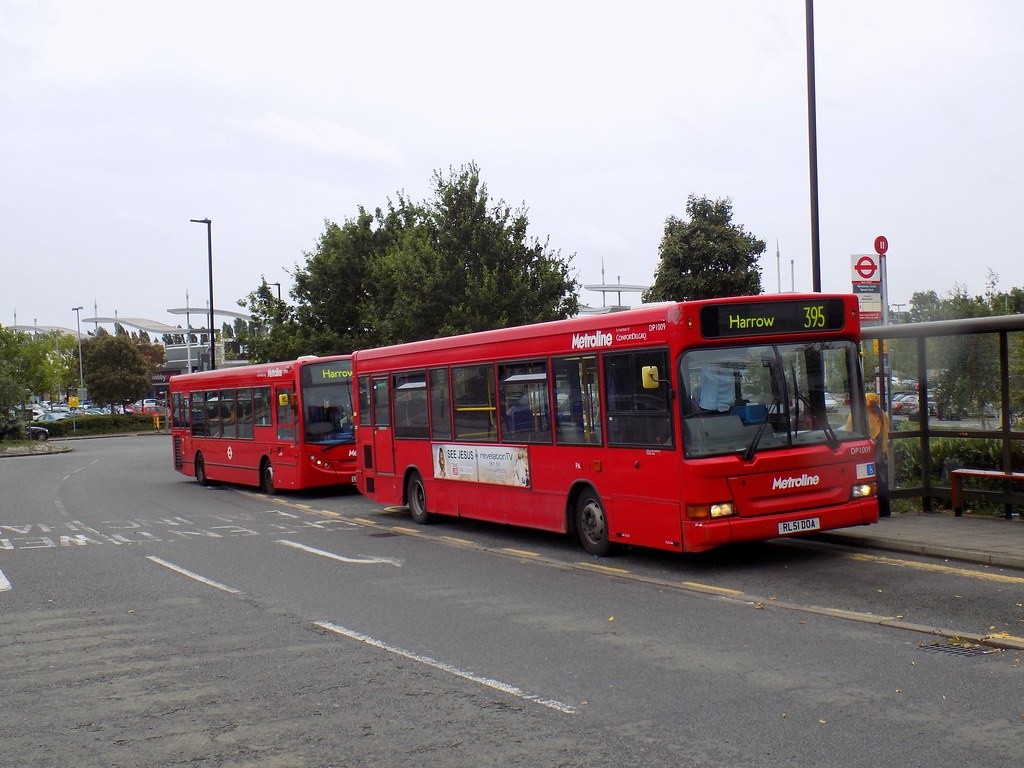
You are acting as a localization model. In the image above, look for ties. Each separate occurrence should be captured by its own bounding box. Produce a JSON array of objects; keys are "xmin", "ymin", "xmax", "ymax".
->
[{"xmin": 732, "ymin": 369, "xmax": 742, "ymax": 402}]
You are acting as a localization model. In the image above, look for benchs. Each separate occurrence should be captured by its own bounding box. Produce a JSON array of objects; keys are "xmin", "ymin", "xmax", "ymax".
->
[
  {"xmin": 684, "ymin": 415, "xmax": 772, "ymax": 446},
  {"xmin": 951, "ymin": 469, "xmax": 1024, "ymax": 517}
]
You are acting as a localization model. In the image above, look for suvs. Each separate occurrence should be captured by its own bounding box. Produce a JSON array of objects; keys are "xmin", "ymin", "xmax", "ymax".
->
[
  {"xmin": 135, "ymin": 406, "xmax": 164, "ymax": 416},
  {"xmin": 135, "ymin": 399, "xmax": 164, "ymax": 407},
  {"xmin": 189, "ymin": 409, "xmax": 210, "ymax": 434},
  {"xmin": 505, "ymin": 386, "xmax": 572, "ymax": 434}
]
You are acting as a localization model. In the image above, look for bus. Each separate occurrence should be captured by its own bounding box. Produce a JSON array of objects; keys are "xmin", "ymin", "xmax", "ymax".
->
[
  {"xmin": 169, "ymin": 356, "xmax": 356, "ymax": 495},
  {"xmin": 351, "ymin": 293, "xmax": 882, "ymax": 556}
]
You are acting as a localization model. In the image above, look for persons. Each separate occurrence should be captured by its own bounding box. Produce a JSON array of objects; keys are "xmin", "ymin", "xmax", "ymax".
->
[
  {"xmin": 691, "ymin": 383, "xmax": 703, "ymax": 414},
  {"xmin": 844, "ymin": 393, "xmax": 892, "ymax": 517},
  {"xmin": 699, "ymin": 350, "xmax": 735, "ymax": 417}
]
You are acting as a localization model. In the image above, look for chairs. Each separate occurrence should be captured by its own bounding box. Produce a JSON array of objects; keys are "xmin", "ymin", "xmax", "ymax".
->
[{"xmin": 512, "ymin": 409, "xmax": 534, "ymax": 440}]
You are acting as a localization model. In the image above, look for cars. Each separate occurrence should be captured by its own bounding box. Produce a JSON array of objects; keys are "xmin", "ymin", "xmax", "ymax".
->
[
  {"xmin": 28, "ymin": 400, "xmax": 134, "ymax": 420},
  {"xmin": 26, "ymin": 426, "xmax": 50, "ymax": 442},
  {"xmin": 824, "ymin": 393, "xmax": 839, "ymax": 412},
  {"xmin": 173, "ymin": 408, "xmax": 190, "ymax": 426},
  {"xmin": 35, "ymin": 414, "xmax": 65, "ymax": 422},
  {"xmin": 877, "ymin": 375, "xmax": 1002, "ymax": 418}
]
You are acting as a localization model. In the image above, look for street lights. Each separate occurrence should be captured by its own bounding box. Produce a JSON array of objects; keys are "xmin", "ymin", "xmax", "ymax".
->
[
  {"xmin": 268, "ymin": 282, "xmax": 281, "ymax": 302},
  {"xmin": 190, "ymin": 217, "xmax": 215, "ymax": 370},
  {"xmin": 892, "ymin": 303, "xmax": 906, "ymax": 325},
  {"xmin": 70, "ymin": 306, "xmax": 85, "ymax": 416}
]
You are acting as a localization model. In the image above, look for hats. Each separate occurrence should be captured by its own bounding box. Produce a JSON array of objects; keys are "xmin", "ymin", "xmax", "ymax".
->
[{"xmin": 865, "ymin": 393, "xmax": 879, "ymax": 406}]
